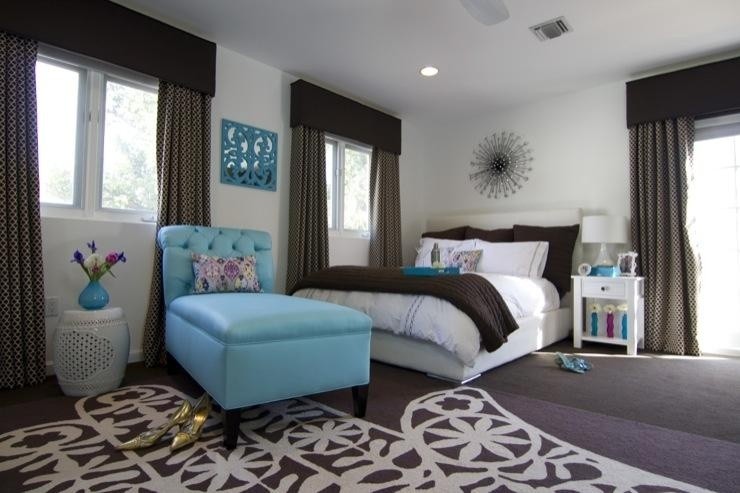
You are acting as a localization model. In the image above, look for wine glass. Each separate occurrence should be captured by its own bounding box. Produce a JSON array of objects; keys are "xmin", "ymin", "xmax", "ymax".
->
[{"xmin": 414, "ymin": 247, "xmax": 430, "ymax": 267}]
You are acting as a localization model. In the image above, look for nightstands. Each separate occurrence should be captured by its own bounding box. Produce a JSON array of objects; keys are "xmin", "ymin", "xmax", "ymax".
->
[{"xmin": 570, "ymin": 275, "xmax": 645, "ymax": 358}]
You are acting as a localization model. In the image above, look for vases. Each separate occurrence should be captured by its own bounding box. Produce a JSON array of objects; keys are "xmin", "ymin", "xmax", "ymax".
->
[
  {"xmin": 70, "ymin": 241, "xmax": 127, "ymax": 281},
  {"xmin": 78, "ymin": 281, "xmax": 110, "ymax": 309}
]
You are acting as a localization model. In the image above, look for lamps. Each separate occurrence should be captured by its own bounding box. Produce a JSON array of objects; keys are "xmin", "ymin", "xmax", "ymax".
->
[{"xmin": 581, "ymin": 215, "xmax": 628, "ymax": 274}]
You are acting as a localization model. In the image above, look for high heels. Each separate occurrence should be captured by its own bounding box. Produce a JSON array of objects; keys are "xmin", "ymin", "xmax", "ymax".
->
[
  {"xmin": 113, "ymin": 399, "xmax": 193, "ymax": 452},
  {"xmin": 566, "ymin": 355, "xmax": 590, "ymax": 370},
  {"xmin": 556, "ymin": 350, "xmax": 584, "ymax": 373},
  {"xmin": 169, "ymin": 391, "xmax": 213, "ymax": 452}
]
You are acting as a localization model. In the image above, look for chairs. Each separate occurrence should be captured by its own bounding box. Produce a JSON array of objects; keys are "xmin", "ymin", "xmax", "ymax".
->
[{"xmin": 158, "ymin": 224, "xmax": 373, "ymax": 448}]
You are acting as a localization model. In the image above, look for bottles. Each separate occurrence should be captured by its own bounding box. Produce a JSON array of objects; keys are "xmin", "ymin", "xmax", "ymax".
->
[{"xmin": 431, "ymin": 243, "xmax": 441, "ymax": 267}]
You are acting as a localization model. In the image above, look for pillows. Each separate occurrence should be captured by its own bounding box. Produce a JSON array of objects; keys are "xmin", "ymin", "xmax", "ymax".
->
[
  {"xmin": 448, "ymin": 250, "xmax": 484, "ymax": 272},
  {"xmin": 416, "ymin": 238, "xmax": 475, "ymax": 252},
  {"xmin": 473, "ymin": 239, "xmax": 549, "ymax": 278},
  {"xmin": 190, "ymin": 253, "xmax": 262, "ymax": 292},
  {"xmin": 415, "ymin": 248, "xmax": 454, "ymax": 267}
]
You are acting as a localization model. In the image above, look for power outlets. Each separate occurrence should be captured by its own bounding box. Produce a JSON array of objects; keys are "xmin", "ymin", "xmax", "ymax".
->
[{"xmin": 43, "ymin": 297, "xmax": 58, "ymax": 316}]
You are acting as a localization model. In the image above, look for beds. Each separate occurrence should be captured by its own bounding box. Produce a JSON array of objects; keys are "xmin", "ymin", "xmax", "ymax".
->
[{"xmin": 292, "ymin": 207, "xmax": 583, "ymax": 385}]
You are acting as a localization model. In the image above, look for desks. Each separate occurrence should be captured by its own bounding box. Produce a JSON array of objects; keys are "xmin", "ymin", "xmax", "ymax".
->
[{"xmin": 52, "ymin": 307, "xmax": 131, "ymax": 396}]
